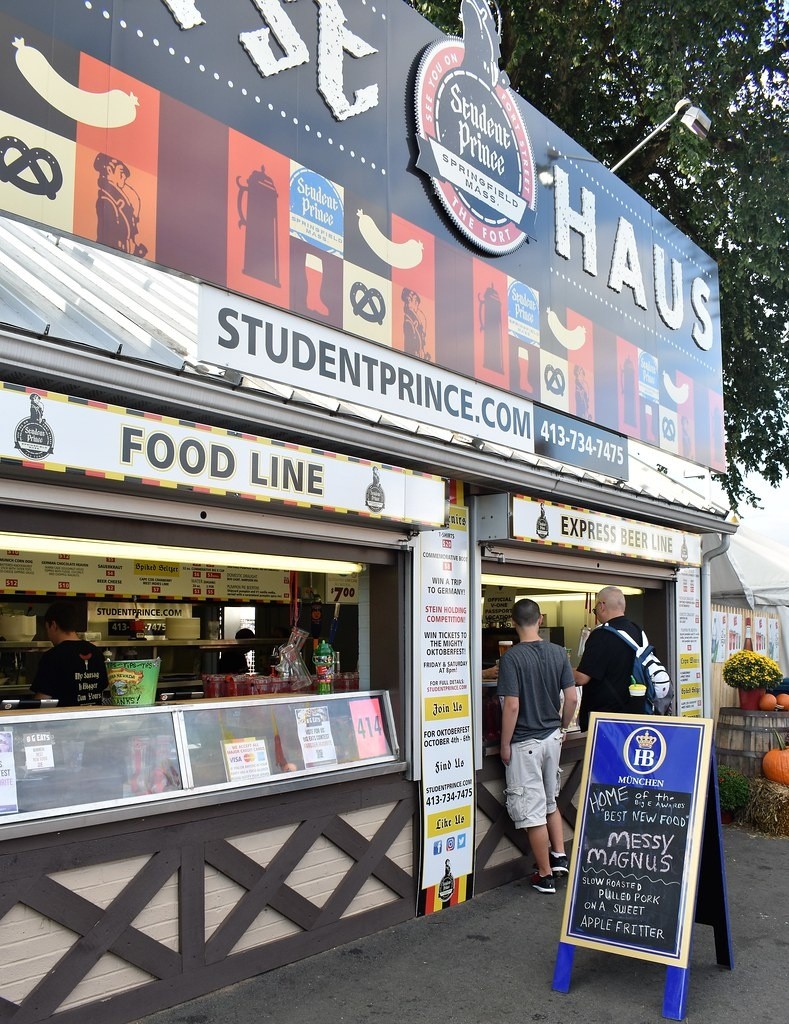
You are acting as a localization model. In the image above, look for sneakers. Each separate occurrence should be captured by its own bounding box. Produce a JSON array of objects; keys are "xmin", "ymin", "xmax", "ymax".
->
[
  {"xmin": 529, "ymin": 871, "xmax": 557, "ymax": 894},
  {"xmin": 532, "ymin": 851, "xmax": 570, "ymax": 878}
]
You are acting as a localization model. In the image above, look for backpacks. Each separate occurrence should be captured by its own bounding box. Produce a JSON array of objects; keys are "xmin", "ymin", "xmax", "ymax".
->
[{"xmin": 604, "ymin": 626, "xmax": 674, "ymax": 716}]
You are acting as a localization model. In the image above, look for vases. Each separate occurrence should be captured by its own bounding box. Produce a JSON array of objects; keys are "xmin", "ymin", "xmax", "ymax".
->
[{"xmin": 739, "ymin": 688, "xmax": 765, "ymax": 711}]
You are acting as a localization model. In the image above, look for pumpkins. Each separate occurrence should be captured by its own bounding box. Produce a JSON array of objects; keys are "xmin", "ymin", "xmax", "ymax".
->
[
  {"xmin": 775, "ymin": 694, "xmax": 789, "ymax": 711},
  {"xmin": 762, "ymin": 728, "xmax": 789, "ymax": 786},
  {"xmin": 759, "ymin": 694, "xmax": 784, "ymax": 711}
]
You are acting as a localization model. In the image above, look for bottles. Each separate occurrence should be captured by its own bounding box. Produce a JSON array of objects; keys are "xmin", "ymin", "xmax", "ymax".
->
[{"xmin": 312, "ymin": 641, "xmax": 334, "ymax": 695}]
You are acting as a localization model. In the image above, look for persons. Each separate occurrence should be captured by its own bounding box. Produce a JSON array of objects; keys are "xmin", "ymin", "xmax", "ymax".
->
[
  {"xmin": 220, "ymin": 629, "xmax": 256, "ymax": 674},
  {"xmin": 500, "ymin": 599, "xmax": 579, "ymax": 894},
  {"xmin": 572, "ymin": 586, "xmax": 650, "ymax": 734},
  {"xmin": 482, "ymin": 662, "xmax": 501, "ymax": 680},
  {"xmin": 29, "ymin": 601, "xmax": 110, "ymax": 708}
]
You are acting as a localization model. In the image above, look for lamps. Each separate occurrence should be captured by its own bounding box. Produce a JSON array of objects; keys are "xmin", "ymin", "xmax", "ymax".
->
[
  {"xmin": 535, "ymin": 148, "xmax": 600, "ymax": 188},
  {"xmin": 610, "ymin": 98, "xmax": 712, "ymax": 173}
]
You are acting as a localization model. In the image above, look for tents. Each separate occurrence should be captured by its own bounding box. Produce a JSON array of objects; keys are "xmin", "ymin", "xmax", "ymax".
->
[{"xmin": 703, "ymin": 525, "xmax": 789, "ymax": 677}]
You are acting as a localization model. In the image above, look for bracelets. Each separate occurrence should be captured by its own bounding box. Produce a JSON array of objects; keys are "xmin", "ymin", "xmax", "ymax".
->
[{"xmin": 560, "ymin": 727, "xmax": 568, "ymax": 733}]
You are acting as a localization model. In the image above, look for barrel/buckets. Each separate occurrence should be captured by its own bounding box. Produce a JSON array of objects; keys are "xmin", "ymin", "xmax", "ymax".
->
[{"xmin": 106, "ymin": 659, "xmax": 163, "ymax": 706}]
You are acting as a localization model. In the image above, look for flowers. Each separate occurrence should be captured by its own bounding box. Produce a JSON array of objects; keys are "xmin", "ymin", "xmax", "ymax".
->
[{"xmin": 723, "ymin": 648, "xmax": 783, "ymax": 690}]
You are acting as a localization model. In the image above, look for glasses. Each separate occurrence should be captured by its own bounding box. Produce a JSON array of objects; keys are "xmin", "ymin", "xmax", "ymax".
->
[{"xmin": 592, "ymin": 602, "xmax": 605, "ymax": 615}]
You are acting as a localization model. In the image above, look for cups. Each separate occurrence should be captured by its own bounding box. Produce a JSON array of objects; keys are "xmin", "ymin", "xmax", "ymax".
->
[
  {"xmin": 279, "ymin": 643, "xmax": 313, "ymax": 686},
  {"xmin": 202, "ymin": 671, "xmax": 359, "ymax": 698},
  {"xmin": 63, "ymin": 740, "xmax": 84, "ymax": 772},
  {"xmin": 276, "ymin": 627, "xmax": 308, "ymax": 672},
  {"xmin": 499, "ymin": 641, "xmax": 513, "ymax": 658}
]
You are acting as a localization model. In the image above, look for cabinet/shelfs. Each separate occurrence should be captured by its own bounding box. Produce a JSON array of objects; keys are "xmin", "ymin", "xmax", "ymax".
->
[{"xmin": 0, "ymin": 636, "xmax": 272, "ymax": 697}]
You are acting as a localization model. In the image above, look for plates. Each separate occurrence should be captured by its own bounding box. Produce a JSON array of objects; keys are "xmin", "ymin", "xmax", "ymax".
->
[{"xmin": 165, "ymin": 617, "xmax": 201, "ymax": 640}]
